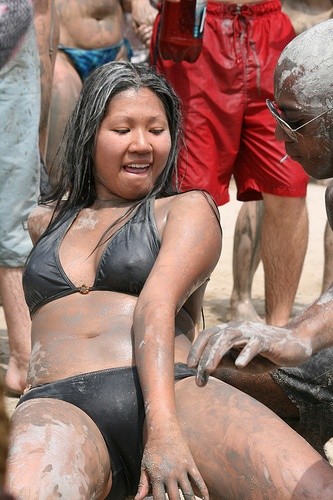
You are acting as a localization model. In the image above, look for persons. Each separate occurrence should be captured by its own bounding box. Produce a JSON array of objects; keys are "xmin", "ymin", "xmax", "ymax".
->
[
  {"xmin": 186, "ymin": 15, "xmax": 333, "ymax": 443},
  {"xmin": 0, "ymin": 59, "xmax": 333, "ymax": 500},
  {"xmin": 0, "ymin": 0, "xmax": 308, "ymax": 395}
]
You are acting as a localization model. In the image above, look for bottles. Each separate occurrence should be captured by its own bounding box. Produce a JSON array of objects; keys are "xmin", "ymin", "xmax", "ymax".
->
[{"xmin": 159, "ymin": 0, "xmax": 207, "ymax": 63}]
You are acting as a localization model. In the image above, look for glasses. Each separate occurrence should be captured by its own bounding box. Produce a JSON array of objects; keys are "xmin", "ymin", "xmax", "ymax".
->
[{"xmin": 266, "ymin": 100, "xmax": 332, "ymax": 141}]
